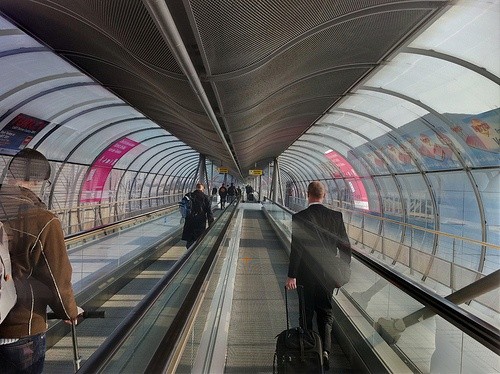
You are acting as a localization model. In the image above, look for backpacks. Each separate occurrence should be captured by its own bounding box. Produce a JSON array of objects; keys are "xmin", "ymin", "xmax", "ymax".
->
[
  {"xmin": 0, "ymin": 204, "xmax": 36, "ymax": 324},
  {"xmin": 178, "ymin": 192, "xmax": 198, "ymax": 218}
]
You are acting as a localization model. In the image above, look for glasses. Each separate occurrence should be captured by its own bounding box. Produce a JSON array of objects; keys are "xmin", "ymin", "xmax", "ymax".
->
[{"xmin": 45, "ymin": 180, "xmax": 52, "ymax": 188}]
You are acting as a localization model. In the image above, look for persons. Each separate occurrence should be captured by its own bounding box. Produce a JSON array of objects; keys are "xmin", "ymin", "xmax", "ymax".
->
[
  {"xmin": 0, "ymin": 147, "xmax": 85, "ymax": 374},
  {"xmin": 181, "ymin": 183, "xmax": 215, "ymax": 249},
  {"xmin": 246, "ymin": 184, "xmax": 254, "ymax": 195},
  {"xmin": 287, "ymin": 181, "xmax": 351, "ymax": 370},
  {"xmin": 218, "ymin": 182, "xmax": 242, "ymax": 210}
]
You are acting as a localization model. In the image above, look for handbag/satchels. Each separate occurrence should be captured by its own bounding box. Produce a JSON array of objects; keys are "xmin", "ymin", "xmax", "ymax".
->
[{"xmin": 323, "ymin": 248, "xmax": 350, "ymax": 290}]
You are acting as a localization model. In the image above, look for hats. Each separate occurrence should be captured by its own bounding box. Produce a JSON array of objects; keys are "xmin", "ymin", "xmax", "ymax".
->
[
  {"xmin": 8, "ymin": 148, "xmax": 51, "ymax": 180},
  {"xmin": 196, "ymin": 182, "xmax": 205, "ymax": 189}
]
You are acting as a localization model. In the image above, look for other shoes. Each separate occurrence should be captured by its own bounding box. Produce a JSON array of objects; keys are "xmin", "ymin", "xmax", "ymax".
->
[{"xmin": 224, "ymin": 207, "xmax": 226, "ymax": 210}]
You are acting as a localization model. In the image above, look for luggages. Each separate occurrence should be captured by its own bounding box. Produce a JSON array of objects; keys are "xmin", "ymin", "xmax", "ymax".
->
[{"xmin": 273, "ymin": 284, "xmax": 323, "ymax": 374}]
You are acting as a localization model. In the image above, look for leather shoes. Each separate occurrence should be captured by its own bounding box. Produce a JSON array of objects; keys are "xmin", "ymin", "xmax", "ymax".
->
[{"xmin": 323, "ymin": 351, "xmax": 330, "ymax": 371}]
